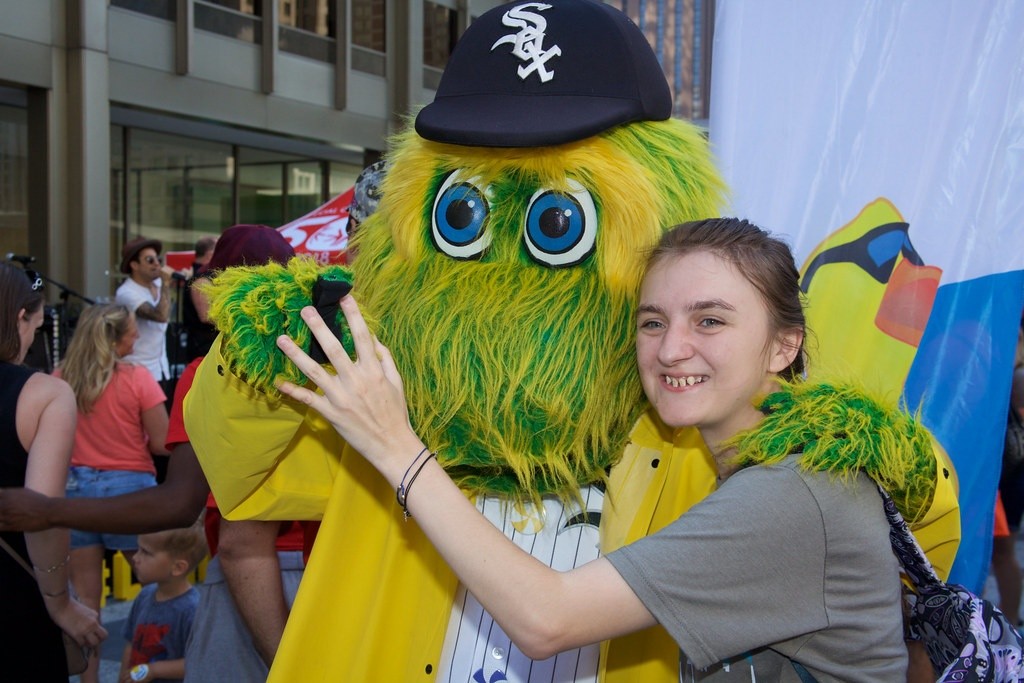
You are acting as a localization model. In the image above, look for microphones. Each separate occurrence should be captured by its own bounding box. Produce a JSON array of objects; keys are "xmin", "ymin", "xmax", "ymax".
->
[
  {"xmin": 6, "ymin": 253, "xmax": 35, "ymax": 263},
  {"xmin": 172, "ymin": 271, "xmax": 189, "ymax": 282}
]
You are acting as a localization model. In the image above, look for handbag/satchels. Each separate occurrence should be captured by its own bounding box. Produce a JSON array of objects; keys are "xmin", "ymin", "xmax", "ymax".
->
[{"xmin": 62, "ymin": 630, "xmax": 88, "ymax": 676}]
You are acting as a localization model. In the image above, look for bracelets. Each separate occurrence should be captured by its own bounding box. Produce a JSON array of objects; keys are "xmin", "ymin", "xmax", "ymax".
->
[{"xmin": 394, "ymin": 447, "xmax": 436, "ymax": 522}]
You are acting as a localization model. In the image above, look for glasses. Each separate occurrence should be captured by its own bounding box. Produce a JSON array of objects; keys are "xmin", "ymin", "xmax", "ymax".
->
[{"xmin": 139, "ymin": 256, "xmax": 164, "ymax": 265}]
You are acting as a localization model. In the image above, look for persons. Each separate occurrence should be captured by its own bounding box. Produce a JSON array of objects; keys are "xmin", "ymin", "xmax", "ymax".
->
[
  {"xmin": 181, "ymin": 238, "xmax": 220, "ymax": 370},
  {"xmin": 344, "ymin": 161, "xmax": 395, "ymax": 268},
  {"xmin": 117, "ymin": 520, "xmax": 208, "ymax": 683},
  {"xmin": 0, "ymin": 224, "xmax": 326, "ymax": 683},
  {"xmin": 0, "ymin": 246, "xmax": 108, "ymax": 682},
  {"xmin": 273, "ymin": 220, "xmax": 918, "ymax": 683},
  {"xmin": 45, "ymin": 298, "xmax": 175, "ymax": 682},
  {"xmin": 111, "ymin": 234, "xmax": 176, "ymax": 486},
  {"xmin": 988, "ymin": 362, "xmax": 1024, "ymax": 625}
]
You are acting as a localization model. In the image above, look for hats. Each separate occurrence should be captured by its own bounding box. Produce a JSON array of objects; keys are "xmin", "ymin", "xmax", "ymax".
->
[
  {"xmin": 120, "ymin": 236, "xmax": 161, "ymax": 276},
  {"xmin": 200, "ymin": 223, "xmax": 295, "ymax": 276}
]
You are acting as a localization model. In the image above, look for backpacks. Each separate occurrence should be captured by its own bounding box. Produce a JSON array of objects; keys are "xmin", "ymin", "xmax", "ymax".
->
[{"xmin": 792, "ymin": 466, "xmax": 1022, "ymax": 683}]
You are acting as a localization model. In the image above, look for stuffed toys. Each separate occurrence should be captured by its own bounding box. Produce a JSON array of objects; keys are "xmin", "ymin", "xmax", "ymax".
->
[{"xmin": 181, "ymin": 0, "xmax": 961, "ymax": 683}]
